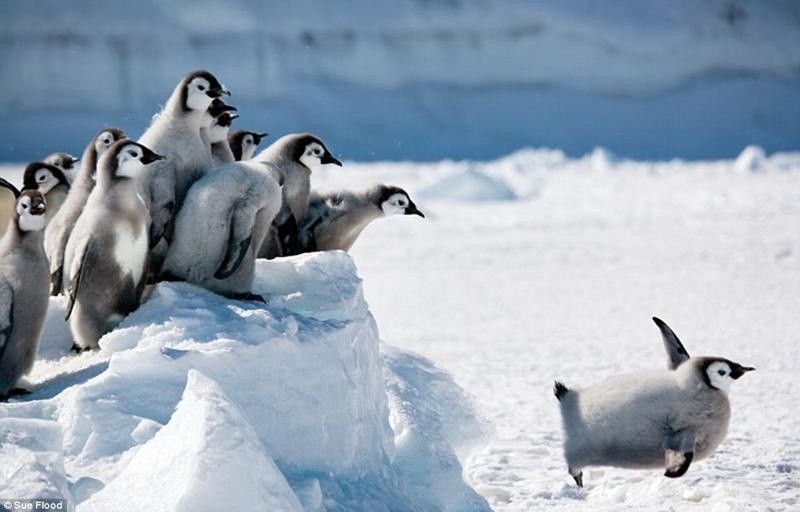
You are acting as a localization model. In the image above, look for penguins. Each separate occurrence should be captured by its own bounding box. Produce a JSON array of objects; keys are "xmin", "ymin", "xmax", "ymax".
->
[
  {"xmin": 0, "ymin": 65, "xmax": 429, "ymax": 402},
  {"xmin": 551, "ymin": 316, "xmax": 756, "ymax": 492}
]
